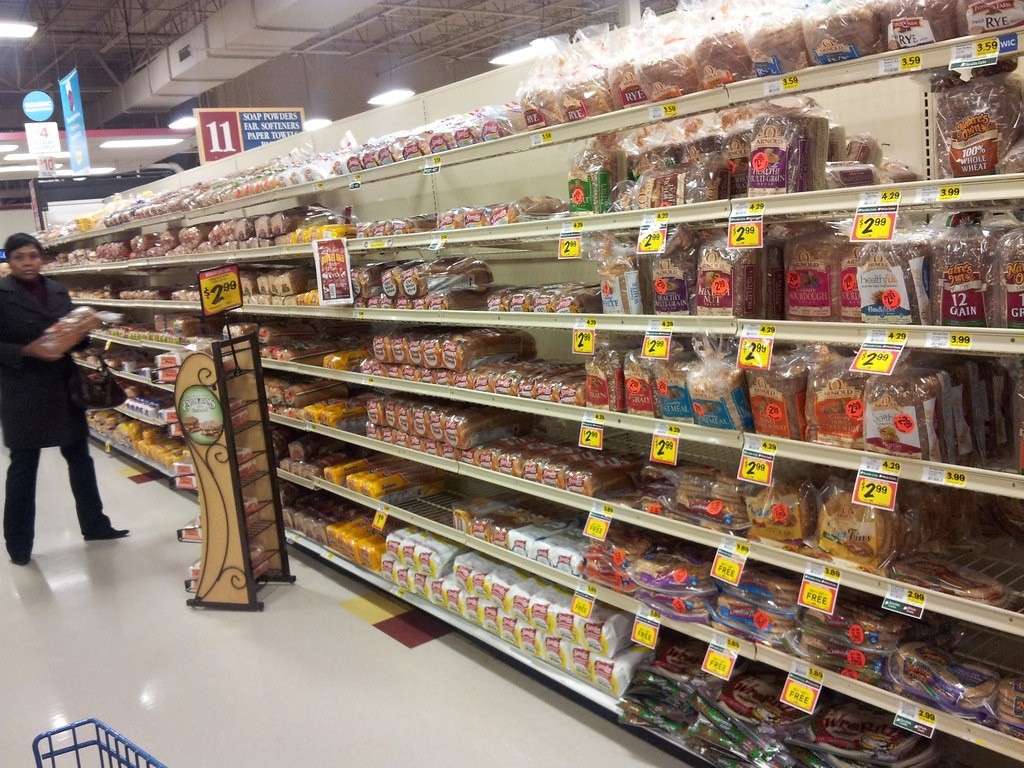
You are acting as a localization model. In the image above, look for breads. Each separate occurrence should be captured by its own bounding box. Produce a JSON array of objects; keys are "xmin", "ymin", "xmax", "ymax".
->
[
  {"xmin": 0, "ymin": 172, "xmax": 260, "ymax": 471},
  {"xmin": 261, "ymin": 0, "xmax": 1024, "ymax": 768}
]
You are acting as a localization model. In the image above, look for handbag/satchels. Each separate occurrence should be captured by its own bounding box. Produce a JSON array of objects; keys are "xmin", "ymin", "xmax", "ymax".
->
[{"xmin": 65, "ymin": 340, "xmax": 128, "ymax": 411}]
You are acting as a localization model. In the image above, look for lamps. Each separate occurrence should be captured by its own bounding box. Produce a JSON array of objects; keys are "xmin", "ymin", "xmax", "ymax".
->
[
  {"xmin": 300, "ymin": 55, "xmax": 333, "ymax": 132},
  {"xmin": 366, "ymin": 35, "xmax": 416, "ymax": 107},
  {"xmin": 0, "ymin": 19, "xmax": 38, "ymax": 38}
]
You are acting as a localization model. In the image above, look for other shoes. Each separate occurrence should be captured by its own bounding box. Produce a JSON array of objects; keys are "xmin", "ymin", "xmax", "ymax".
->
[
  {"xmin": 84, "ymin": 527, "xmax": 129, "ymax": 541},
  {"xmin": 12, "ymin": 556, "xmax": 30, "ymax": 565}
]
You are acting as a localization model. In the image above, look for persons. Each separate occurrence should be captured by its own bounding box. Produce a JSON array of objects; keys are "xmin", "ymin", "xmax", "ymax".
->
[{"xmin": 1, "ymin": 233, "xmax": 131, "ymax": 567}]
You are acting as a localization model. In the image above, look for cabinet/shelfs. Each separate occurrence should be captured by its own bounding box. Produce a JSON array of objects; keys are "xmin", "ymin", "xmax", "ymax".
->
[
  {"xmin": 147, "ymin": 332, "xmax": 297, "ymax": 612},
  {"xmin": 0, "ymin": 0, "xmax": 1024, "ymax": 768}
]
